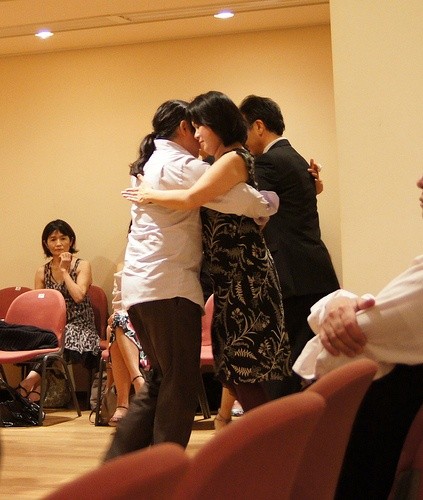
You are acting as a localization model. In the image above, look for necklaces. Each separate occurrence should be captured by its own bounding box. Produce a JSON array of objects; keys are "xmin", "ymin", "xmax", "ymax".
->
[{"xmin": 51, "ymin": 274, "xmax": 58, "ymax": 283}]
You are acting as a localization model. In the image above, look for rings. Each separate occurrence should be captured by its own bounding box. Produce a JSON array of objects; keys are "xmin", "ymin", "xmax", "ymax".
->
[{"xmin": 317, "ymin": 164, "xmax": 322, "ymax": 171}]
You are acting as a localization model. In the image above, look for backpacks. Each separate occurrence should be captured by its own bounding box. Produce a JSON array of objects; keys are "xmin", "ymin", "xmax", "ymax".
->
[{"xmin": 90, "ymin": 373, "xmax": 106, "ymax": 411}]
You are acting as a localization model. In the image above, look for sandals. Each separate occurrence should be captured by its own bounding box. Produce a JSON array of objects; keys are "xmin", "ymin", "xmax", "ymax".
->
[
  {"xmin": 130, "ymin": 376, "xmax": 147, "ymax": 393},
  {"xmin": 108, "ymin": 406, "xmax": 129, "ymax": 426},
  {"xmin": 214, "ymin": 414, "xmax": 230, "ymax": 433}
]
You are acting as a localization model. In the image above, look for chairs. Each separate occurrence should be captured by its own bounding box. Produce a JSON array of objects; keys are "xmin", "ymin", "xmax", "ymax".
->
[{"xmin": 0, "ymin": 282, "xmax": 422, "ymax": 500}]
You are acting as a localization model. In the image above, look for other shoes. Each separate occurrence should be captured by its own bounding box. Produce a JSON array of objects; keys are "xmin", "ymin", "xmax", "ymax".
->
[
  {"xmin": 26, "ymin": 391, "xmax": 42, "ymax": 405},
  {"xmin": 231, "ymin": 409, "xmax": 243, "ymax": 416},
  {"xmin": 14, "ymin": 384, "xmax": 31, "ymax": 399}
]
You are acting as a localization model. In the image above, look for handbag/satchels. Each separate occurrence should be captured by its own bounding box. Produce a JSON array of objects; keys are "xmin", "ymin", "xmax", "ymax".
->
[
  {"xmin": 42, "ymin": 367, "xmax": 69, "ymax": 407},
  {"xmin": 90, "ymin": 383, "xmax": 118, "ymax": 426}
]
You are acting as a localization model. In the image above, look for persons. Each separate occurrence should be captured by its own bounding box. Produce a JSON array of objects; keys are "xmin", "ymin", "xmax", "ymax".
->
[
  {"xmin": 119, "ymin": 89, "xmax": 291, "ymax": 415},
  {"xmin": 14, "ymin": 219, "xmax": 103, "ymax": 408},
  {"xmin": 99, "ymin": 100, "xmax": 278, "ymax": 468},
  {"xmin": 106, "ymin": 220, "xmax": 146, "ymax": 425},
  {"xmin": 211, "ymin": 94, "xmax": 340, "ymax": 434},
  {"xmin": 291, "ymin": 178, "xmax": 422, "ymax": 500}
]
